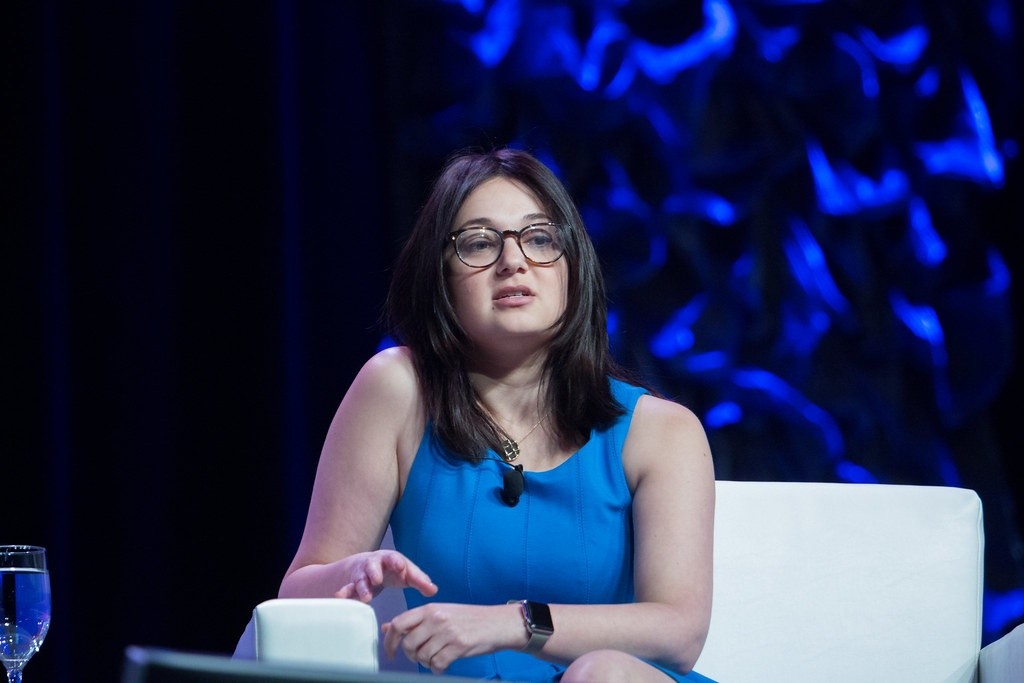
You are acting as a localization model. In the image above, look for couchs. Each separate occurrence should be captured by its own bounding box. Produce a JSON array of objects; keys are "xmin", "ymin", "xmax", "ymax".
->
[{"xmin": 232, "ymin": 480, "xmax": 1024, "ymax": 681}]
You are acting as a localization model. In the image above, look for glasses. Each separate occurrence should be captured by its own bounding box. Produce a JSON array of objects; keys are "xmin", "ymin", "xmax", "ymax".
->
[{"xmin": 445, "ymin": 223, "xmax": 567, "ymax": 267}]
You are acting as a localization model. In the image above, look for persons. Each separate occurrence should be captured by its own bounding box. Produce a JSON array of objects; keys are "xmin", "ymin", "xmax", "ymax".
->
[{"xmin": 278, "ymin": 148, "xmax": 717, "ymax": 683}]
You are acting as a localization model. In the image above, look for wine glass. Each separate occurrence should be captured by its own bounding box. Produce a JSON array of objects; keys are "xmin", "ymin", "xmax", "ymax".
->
[{"xmin": 0, "ymin": 545, "xmax": 53, "ymax": 683}]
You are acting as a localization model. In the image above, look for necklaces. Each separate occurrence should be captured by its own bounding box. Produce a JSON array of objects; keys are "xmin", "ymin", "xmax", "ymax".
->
[{"xmin": 491, "ymin": 409, "xmax": 548, "ymax": 461}]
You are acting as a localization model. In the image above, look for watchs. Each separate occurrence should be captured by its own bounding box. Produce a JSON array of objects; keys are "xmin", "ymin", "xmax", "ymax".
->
[{"xmin": 506, "ymin": 600, "xmax": 553, "ymax": 653}]
